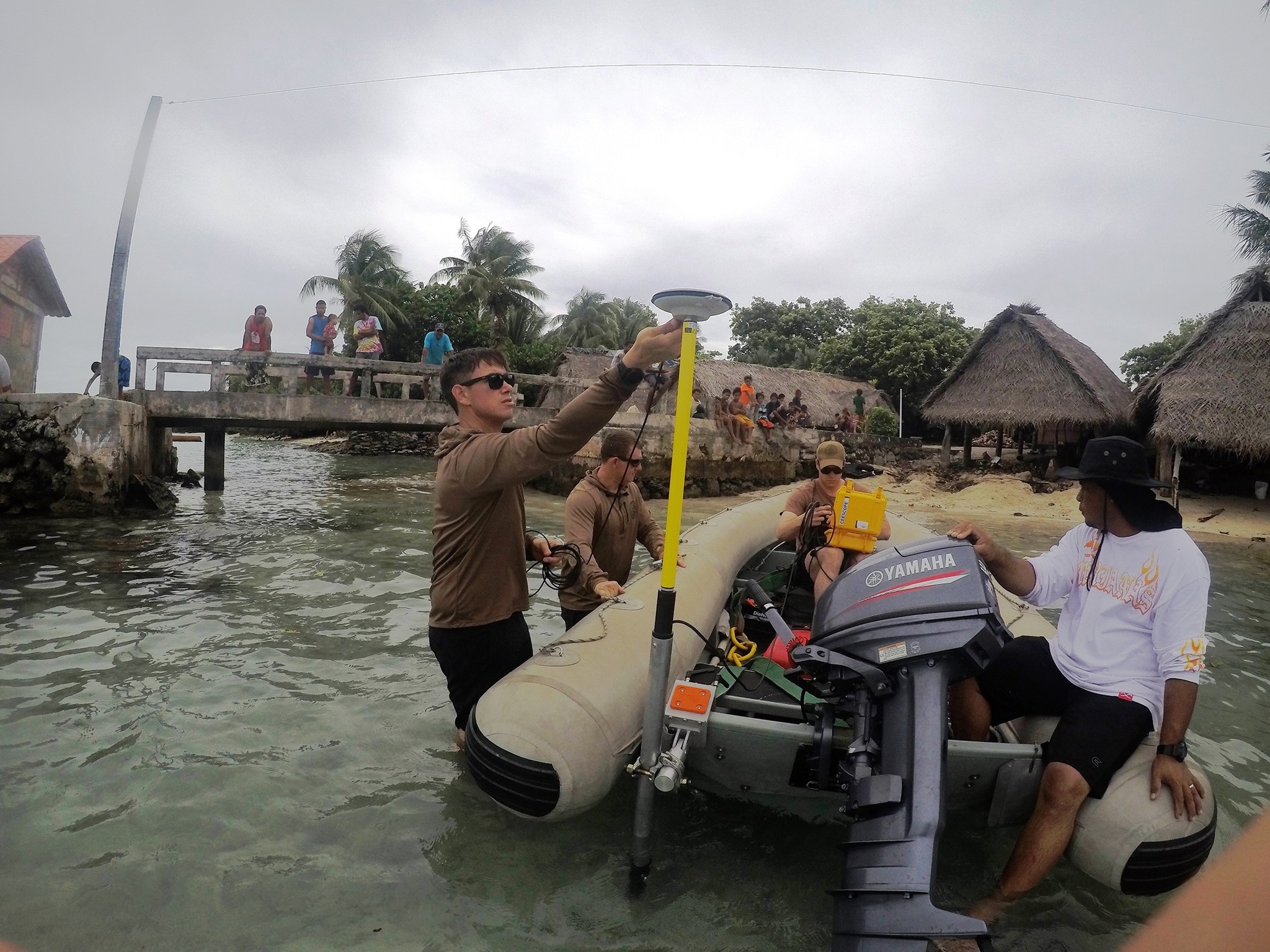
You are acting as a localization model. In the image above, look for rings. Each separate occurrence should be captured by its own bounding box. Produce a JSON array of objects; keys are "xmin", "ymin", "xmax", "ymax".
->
[{"xmin": 1189, "ymin": 783, "xmax": 1195, "ymax": 788}]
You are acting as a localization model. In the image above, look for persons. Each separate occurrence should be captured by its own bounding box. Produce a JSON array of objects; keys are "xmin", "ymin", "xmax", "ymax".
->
[
  {"xmin": 774, "ymin": 441, "xmax": 891, "ymax": 608},
  {"xmin": 1111, "ymin": 800, "xmax": 1270, "ymax": 951},
  {"xmin": 303, "ymin": 299, "xmax": 338, "ymax": 397},
  {"xmin": 714, "ymin": 375, "xmax": 811, "ymax": 446},
  {"xmin": 835, "ymin": 388, "xmax": 866, "ymax": 434},
  {"xmin": 558, "ymin": 430, "xmax": 686, "ymax": 632},
  {"xmin": 84, "ymin": 354, "xmax": 131, "ymax": 398},
  {"xmin": 421, "ymin": 322, "xmax": 454, "ymax": 400},
  {"xmin": 0, "ymin": 354, "xmax": 12, "ymax": 394},
  {"xmin": 946, "ymin": 436, "xmax": 1207, "ymax": 928},
  {"xmin": 690, "ymin": 388, "xmax": 706, "ymax": 419},
  {"xmin": 429, "ymin": 318, "xmax": 683, "ymax": 753},
  {"xmin": 610, "ymin": 339, "xmax": 673, "ymax": 414},
  {"xmin": 241, "ymin": 305, "xmax": 273, "ymax": 393},
  {"xmin": 340, "ymin": 303, "xmax": 383, "ymax": 396}
]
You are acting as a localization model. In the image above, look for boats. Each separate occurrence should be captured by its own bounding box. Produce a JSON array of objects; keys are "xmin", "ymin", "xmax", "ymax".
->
[{"xmin": 463, "ymin": 487, "xmax": 1218, "ymax": 952}]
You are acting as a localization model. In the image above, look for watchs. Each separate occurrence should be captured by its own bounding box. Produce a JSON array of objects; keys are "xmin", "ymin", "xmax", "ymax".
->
[
  {"xmin": 1156, "ymin": 740, "xmax": 1188, "ymax": 762},
  {"xmin": 617, "ymin": 359, "xmax": 645, "ymax": 384}
]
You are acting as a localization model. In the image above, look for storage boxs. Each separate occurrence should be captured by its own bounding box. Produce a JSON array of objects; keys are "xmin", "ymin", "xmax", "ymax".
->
[{"xmin": 824, "ymin": 479, "xmax": 888, "ymax": 554}]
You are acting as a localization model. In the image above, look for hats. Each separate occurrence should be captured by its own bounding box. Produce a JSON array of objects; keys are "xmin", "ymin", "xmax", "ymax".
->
[
  {"xmin": 816, "ymin": 440, "xmax": 846, "ymax": 470},
  {"xmin": 435, "ymin": 323, "xmax": 445, "ymax": 331},
  {"xmin": 1056, "ymin": 437, "xmax": 1174, "ymax": 489}
]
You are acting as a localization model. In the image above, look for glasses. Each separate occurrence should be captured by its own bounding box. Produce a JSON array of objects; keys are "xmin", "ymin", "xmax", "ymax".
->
[
  {"xmin": 817, "ymin": 461, "xmax": 844, "ymax": 474},
  {"xmin": 459, "ymin": 373, "xmax": 515, "ymax": 390},
  {"xmin": 610, "ymin": 455, "xmax": 644, "ymax": 467}
]
